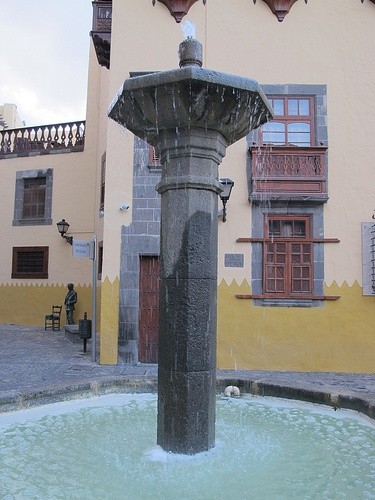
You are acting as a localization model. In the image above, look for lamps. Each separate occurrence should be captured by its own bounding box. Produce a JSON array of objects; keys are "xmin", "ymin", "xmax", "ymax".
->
[
  {"xmin": 56, "ymin": 219, "xmax": 73, "ymax": 246},
  {"xmin": 218, "ymin": 177, "xmax": 234, "ymax": 222}
]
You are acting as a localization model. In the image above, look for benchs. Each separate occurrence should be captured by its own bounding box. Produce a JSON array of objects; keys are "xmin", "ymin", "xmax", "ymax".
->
[{"xmin": 63, "ymin": 324, "xmax": 85, "ymax": 344}]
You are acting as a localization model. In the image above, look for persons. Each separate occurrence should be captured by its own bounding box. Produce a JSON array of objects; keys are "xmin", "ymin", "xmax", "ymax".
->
[{"xmin": 63, "ymin": 282, "xmax": 78, "ymax": 325}]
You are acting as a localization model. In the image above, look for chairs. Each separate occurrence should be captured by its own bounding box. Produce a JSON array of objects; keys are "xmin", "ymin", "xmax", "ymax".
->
[{"xmin": 44, "ymin": 305, "xmax": 62, "ymax": 332}]
